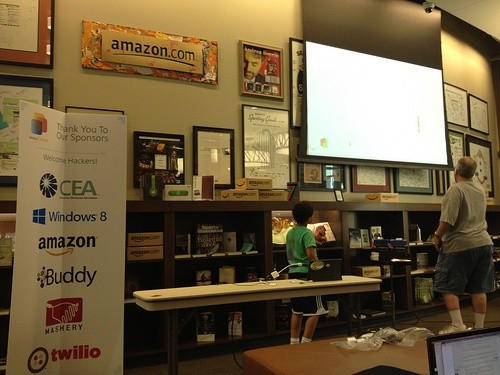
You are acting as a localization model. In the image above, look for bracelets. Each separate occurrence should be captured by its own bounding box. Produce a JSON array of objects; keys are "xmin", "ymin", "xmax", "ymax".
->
[{"xmin": 434, "ymin": 231, "xmax": 442, "ymax": 239}]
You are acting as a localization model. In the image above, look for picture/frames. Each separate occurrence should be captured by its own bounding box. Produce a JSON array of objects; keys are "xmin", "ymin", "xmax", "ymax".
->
[
  {"xmin": 133, "ymin": 130, "xmax": 185, "ymax": 189},
  {"xmin": 290, "ymin": 37, "xmax": 495, "ymax": 198},
  {"xmin": 238, "ymin": 40, "xmax": 284, "ymax": 101},
  {"xmin": 242, "ymin": 103, "xmax": 291, "ymax": 190},
  {"xmin": 0, "ymin": 73, "xmax": 56, "ymax": 186},
  {"xmin": 192, "ymin": 126, "xmax": 236, "ymax": 189},
  {"xmin": 0, "ymin": 0, "xmax": 54, "ymax": 69}
]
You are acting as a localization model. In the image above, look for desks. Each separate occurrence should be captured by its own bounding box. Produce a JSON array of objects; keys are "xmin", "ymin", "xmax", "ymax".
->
[
  {"xmin": 243, "ymin": 330, "xmax": 434, "ymax": 375},
  {"xmin": 133, "ymin": 275, "xmax": 382, "ymax": 375}
]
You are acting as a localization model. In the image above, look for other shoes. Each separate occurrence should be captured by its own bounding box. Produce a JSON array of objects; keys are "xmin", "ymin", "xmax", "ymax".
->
[
  {"xmin": 468, "ymin": 327, "xmax": 485, "ymax": 331},
  {"xmin": 439, "ymin": 324, "xmax": 467, "ymax": 334}
]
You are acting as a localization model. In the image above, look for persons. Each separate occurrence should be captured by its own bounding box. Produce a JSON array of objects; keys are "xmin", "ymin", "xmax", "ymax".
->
[
  {"xmin": 286, "ymin": 200, "xmax": 330, "ymax": 344},
  {"xmin": 243, "ymin": 45, "xmax": 269, "ymax": 84},
  {"xmin": 434, "ymin": 156, "xmax": 496, "ymax": 334}
]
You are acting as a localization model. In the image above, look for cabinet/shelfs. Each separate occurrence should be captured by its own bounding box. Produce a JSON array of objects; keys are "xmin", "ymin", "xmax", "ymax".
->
[{"xmin": 0, "ymin": 201, "xmax": 500, "ymax": 375}]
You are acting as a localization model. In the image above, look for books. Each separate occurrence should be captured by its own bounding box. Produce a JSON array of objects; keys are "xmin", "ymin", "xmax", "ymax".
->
[
  {"xmin": 349, "ymin": 226, "xmax": 408, "ymax": 260},
  {"xmin": 195, "ymin": 312, "xmax": 215, "ymax": 342},
  {"xmin": 414, "ymin": 277, "xmax": 434, "ymax": 305},
  {"xmin": 381, "ymin": 292, "xmax": 395, "ymax": 312},
  {"xmin": 228, "ymin": 312, "xmax": 243, "ymax": 337}
]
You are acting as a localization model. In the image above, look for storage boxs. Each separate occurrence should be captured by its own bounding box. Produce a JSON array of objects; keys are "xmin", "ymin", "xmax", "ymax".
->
[
  {"xmin": 351, "ymin": 265, "xmax": 381, "ymax": 278},
  {"xmin": 127, "ymin": 231, "xmax": 163, "ymax": 247},
  {"xmin": 127, "ymin": 246, "xmax": 164, "ymax": 262},
  {"xmin": 221, "ymin": 179, "xmax": 289, "ymax": 201}
]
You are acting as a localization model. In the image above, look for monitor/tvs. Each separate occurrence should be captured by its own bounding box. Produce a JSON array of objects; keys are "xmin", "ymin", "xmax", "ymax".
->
[{"xmin": 426, "ymin": 326, "xmax": 500, "ymax": 375}]
